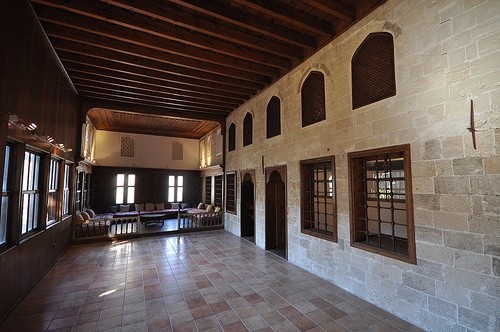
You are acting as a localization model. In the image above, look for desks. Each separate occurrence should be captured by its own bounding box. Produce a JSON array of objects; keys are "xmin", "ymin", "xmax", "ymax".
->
[{"xmin": 139, "ymin": 213, "xmax": 166, "ymax": 226}]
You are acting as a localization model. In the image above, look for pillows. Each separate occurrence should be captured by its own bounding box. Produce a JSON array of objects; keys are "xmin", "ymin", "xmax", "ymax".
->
[
  {"xmin": 196, "ymin": 202, "xmax": 203, "ymax": 209},
  {"xmin": 206, "ymin": 205, "xmax": 212, "ymax": 212},
  {"xmin": 171, "ymin": 203, "xmax": 179, "ymax": 209},
  {"xmin": 180, "ymin": 203, "xmax": 190, "ymax": 208},
  {"xmin": 156, "ymin": 203, "xmax": 165, "ymax": 210},
  {"xmin": 135, "ymin": 204, "xmax": 144, "ymax": 211},
  {"xmin": 75, "ymin": 214, "xmax": 84, "ymax": 225},
  {"xmin": 144, "ymin": 202, "xmax": 155, "ymax": 211},
  {"xmin": 107, "ymin": 206, "xmax": 118, "ymax": 212},
  {"xmin": 212, "ymin": 207, "xmax": 221, "ymax": 216},
  {"xmin": 81, "ymin": 211, "xmax": 90, "ymax": 220},
  {"xmin": 86, "ymin": 208, "xmax": 95, "ymax": 217},
  {"xmin": 120, "ymin": 204, "xmax": 130, "ymax": 212}
]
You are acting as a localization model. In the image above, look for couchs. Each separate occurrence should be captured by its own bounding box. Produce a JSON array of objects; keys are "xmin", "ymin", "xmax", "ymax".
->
[{"xmin": 73, "ymin": 204, "xmax": 221, "ymax": 239}]
